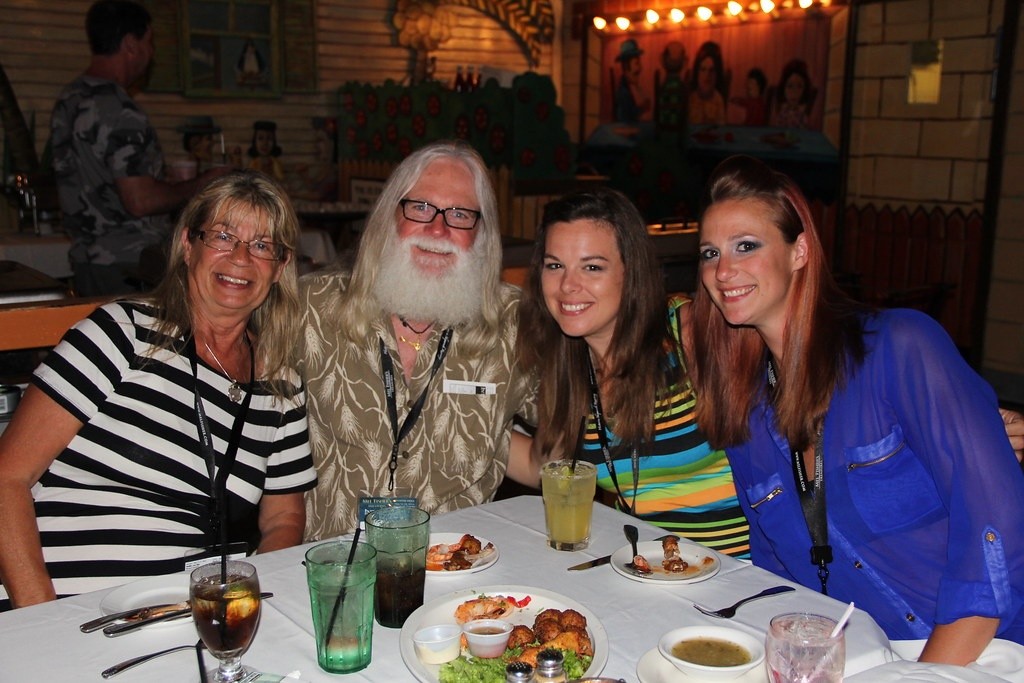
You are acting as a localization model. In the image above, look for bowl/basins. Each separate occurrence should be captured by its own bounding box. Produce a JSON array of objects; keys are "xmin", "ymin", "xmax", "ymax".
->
[{"xmin": 658, "ymin": 625, "xmax": 766, "ymax": 681}]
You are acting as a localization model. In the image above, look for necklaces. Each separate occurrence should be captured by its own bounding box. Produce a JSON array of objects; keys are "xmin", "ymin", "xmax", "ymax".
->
[
  {"xmin": 200, "ymin": 336, "xmax": 241, "ymax": 403},
  {"xmin": 398, "ymin": 315, "xmax": 436, "ymax": 350}
]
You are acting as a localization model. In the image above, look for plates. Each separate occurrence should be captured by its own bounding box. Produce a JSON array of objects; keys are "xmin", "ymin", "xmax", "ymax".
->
[
  {"xmin": 100, "ymin": 575, "xmax": 194, "ymax": 631},
  {"xmin": 610, "ymin": 541, "xmax": 721, "ymax": 585},
  {"xmin": 636, "ymin": 646, "xmax": 768, "ymax": 683},
  {"xmin": 399, "ymin": 585, "xmax": 609, "ymax": 683},
  {"xmin": 425, "ymin": 532, "xmax": 500, "ymax": 577}
]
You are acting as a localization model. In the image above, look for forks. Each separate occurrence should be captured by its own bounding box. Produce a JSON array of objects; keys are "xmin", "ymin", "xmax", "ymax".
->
[{"xmin": 693, "ymin": 585, "xmax": 795, "ymax": 618}]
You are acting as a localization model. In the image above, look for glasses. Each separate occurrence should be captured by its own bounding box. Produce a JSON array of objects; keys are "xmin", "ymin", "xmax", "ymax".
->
[
  {"xmin": 195, "ymin": 230, "xmax": 285, "ymax": 260},
  {"xmin": 398, "ymin": 198, "xmax": 481, "ymax": 230}
]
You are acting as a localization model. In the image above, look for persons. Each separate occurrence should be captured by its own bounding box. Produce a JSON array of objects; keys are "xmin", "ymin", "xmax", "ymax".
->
[
  {"xmin": 43, "ymin": 0, "xmax": 225, "ymax": 295},
  {"xmin": 284, "ymin": 144, "xmax": 540, "ymax": 542},
  {"xmin": 507, "ymin": 188, "xmax": 1024, "ymax": 565},
  {"xmin": 0, "ymin": 176, "xmax": 320, "ymax": 613},
  {"xmin": 690, "ymin": 155, "xmax": 1024, "ymax": 683}
]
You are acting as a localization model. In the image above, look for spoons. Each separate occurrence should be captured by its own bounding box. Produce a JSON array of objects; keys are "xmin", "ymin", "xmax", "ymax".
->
[{"xmin": 623, "ymin": 525, "xmax": 653, "ymax": 577}]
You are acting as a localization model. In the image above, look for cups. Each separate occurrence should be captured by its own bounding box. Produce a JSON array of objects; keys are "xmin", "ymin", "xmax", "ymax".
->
[
  {"xmin": 412, "ymin": 618, "xmax": 514, "ymax": 664},
  {"xmin": 765, "ymin": 612, "xmax": 845, "ymax": 683},
  {"xmin": 539, "ymin": 459, "xmax": 596, "ymax": 552},
  {"xmin": 305, "ymin": 506, "xmax": 430, "ymax": 674}
]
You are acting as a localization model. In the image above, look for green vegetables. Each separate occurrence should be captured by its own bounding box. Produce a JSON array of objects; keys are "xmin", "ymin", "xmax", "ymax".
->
[{"xmin": 436, "ymin": 639, "xmax": 594, "ymax": 683}]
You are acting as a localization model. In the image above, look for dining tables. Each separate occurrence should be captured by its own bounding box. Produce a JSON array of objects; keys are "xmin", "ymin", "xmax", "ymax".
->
[
  {"xmin": 0, "ymin": 228, "xmax": 76, "ymax": 291},
  {"xmin": 0, "ymin": 494, "xmax": 892, "ymax": 683}
]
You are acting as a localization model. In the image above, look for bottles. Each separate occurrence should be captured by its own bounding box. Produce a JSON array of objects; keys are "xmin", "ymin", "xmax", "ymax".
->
[{"xmin": 504, "ymin": 649, "xmax": 568, "ymax": 683}]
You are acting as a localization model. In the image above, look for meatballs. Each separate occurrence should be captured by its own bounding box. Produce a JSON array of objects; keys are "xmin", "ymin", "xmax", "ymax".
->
[
  {"xmin": 461, "ymin": 536, "xmax": 482, "ymax": 555},
  {"xmin": 508, "ymin": 608, "xmax": 589, "ymax": 650}
]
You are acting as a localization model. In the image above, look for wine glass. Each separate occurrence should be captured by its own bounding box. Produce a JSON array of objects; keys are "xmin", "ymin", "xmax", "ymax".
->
[{"xmin": 188, "ymin": 559, "xmax": 261, "ymax": 683}]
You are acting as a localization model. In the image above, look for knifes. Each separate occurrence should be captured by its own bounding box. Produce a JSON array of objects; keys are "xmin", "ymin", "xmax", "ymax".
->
[
  {"xmin": 567, "ymin": 534, "xmax": 679, "ymax": 572},
  {"xmin": 104, "ymin": 592, "xmax": 274, "ymax": 638}
]
